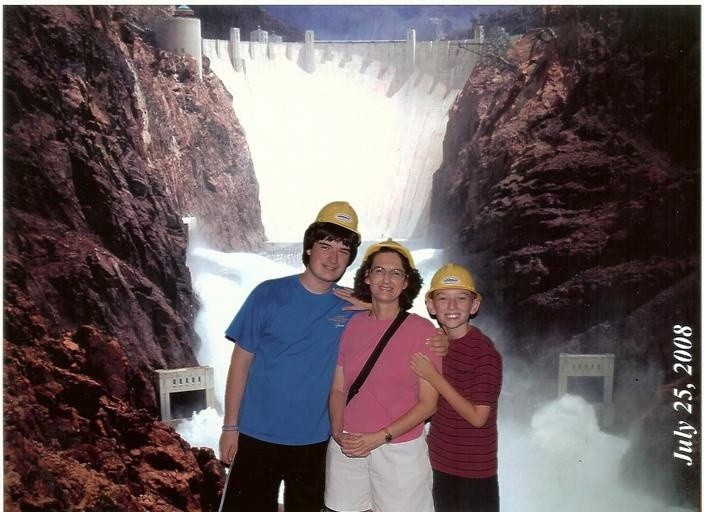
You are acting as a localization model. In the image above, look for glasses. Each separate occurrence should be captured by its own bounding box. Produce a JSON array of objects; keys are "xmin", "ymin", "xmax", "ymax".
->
[{"xmin": 368, "ymin": 265, "xmax": 408, "ymax": 277}]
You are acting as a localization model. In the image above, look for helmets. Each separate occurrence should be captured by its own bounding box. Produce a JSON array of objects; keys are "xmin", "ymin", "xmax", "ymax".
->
[
  {"xmin": 315, "ymin": 201, "xmax": 360, "ymax": 236},
  {"xmin": 363, "ymin": 236, "xmax": 416, "ymax": 271},
  {"xmin": 430, "ymin": 264, "xmax": 478, "ymax": 296}
]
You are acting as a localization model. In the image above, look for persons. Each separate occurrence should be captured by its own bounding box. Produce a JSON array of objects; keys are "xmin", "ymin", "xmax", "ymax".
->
[
  {"xmin": 215, "ymin": 199, "xmax": 450, "ymax": 512},
  {"xmin": 329, "ymin": 259, "xmax": 503, "ymax": 512},
  {"xmin": 321, "ymin": 237, "xmax": 444, "ymax": 512}
]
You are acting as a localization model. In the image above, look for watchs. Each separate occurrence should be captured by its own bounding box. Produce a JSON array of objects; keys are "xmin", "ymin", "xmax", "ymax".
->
[{"xmin": 381, "ymin": 427, "xmax": 393, "ymax": 446}]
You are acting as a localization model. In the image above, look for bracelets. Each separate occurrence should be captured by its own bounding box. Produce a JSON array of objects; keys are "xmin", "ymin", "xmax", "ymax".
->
[{"xmin": 220, "ymin": 425, "xmax": 240, "ymax": 433}]
